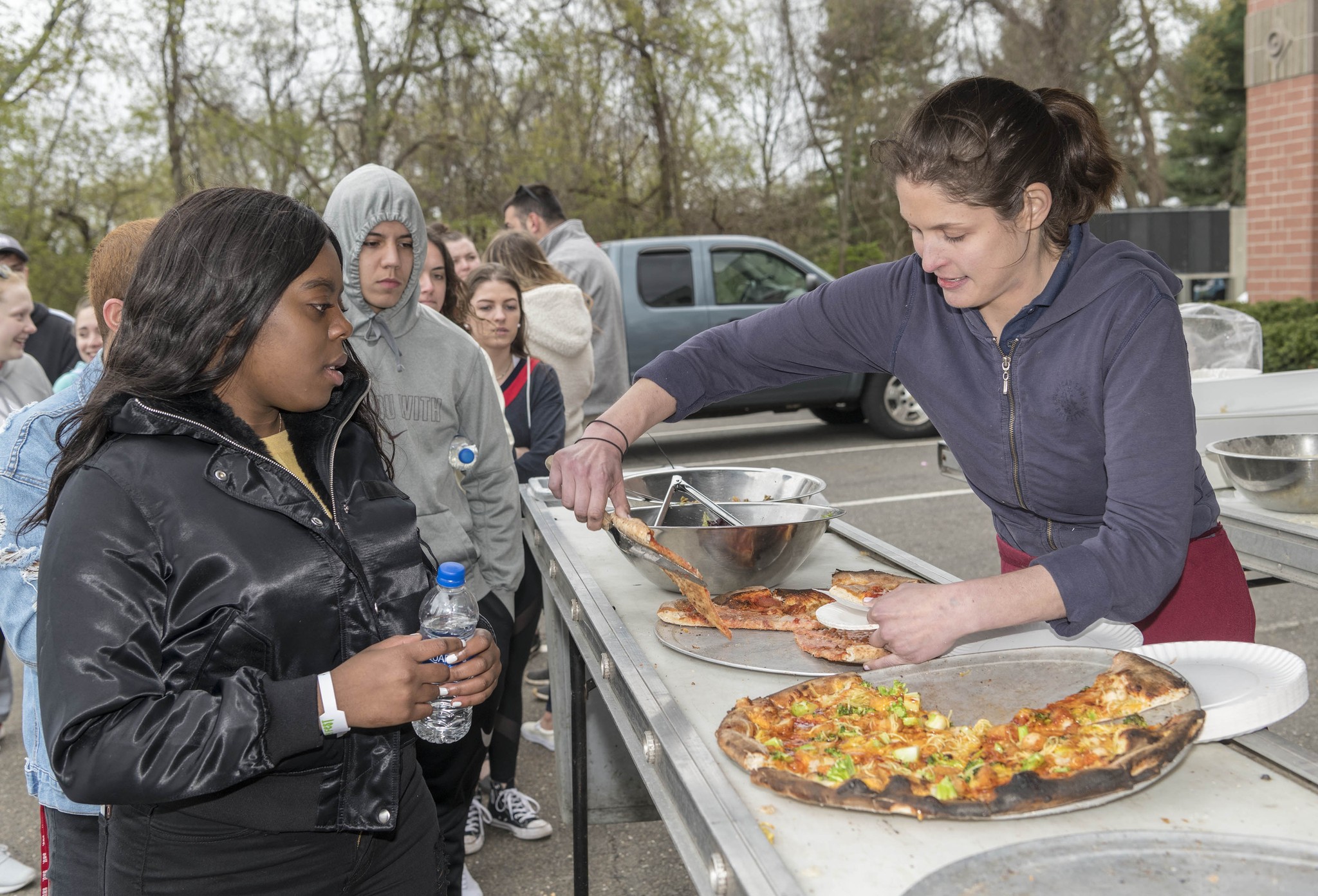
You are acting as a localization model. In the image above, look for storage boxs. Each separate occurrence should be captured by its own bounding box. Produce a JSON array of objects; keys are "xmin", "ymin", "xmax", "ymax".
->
[{"xmin": 1191, "ymin": 367, "xmax": 1318, "ymax": 488}]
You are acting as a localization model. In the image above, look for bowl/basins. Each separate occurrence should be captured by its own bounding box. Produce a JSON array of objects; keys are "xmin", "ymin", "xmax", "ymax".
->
[
  {"xmin": 606, "ymin": 502, "xmax": 847, "ymax": 594},
  {"xmin": 607, "ymin": 467, "xmax": 826, "ymax": 507},
  {"xmin": 1205, "ymin": 434, "xmax": 1317, "ymax": 515}
]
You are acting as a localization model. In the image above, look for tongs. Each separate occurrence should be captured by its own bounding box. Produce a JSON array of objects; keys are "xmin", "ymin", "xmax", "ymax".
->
[
  {"xmin": 654, "ymin": 475, "xmax": 746, "ymax": 527},
  {"xmin": 625, "ymin": 488, "xmax": 663, "ymax": 502}
]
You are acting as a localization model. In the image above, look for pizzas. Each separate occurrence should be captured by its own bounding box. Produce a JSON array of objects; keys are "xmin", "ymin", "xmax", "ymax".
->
[
  {"xmin": 716, "ymin": 650, "xmax": 1205, "ymax": 818},
  {"xmin": 609, "ymin": 512, "xmax": 925, "ymax": 663}
]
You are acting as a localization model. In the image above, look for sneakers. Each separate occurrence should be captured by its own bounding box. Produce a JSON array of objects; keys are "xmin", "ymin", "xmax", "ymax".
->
[
  {"xmin": 525, "ymin": 669, "xmax": 550, "ymax": 686},
  {"xmin": 486, "ymin": 781, "xmax": 553, "ymax": 840},
  {"xmin": 463, "ymin": 784, "xmax": 492, "ymax": 853},
  {"xmin": 533, "ymin": 684, "xmax": 550, "ymax": 701},
  {"xmin": 0, "ymin": 844, "xmax": 37, "ymax": 894},
  {"xmin": 520, "ymin": 719, "xmax": 556, "ymax": 751}
]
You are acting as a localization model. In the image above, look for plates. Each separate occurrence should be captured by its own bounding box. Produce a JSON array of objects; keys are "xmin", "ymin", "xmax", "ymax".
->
[
  {"xmin": 938, "ymin": 617, "xmax": 1145, "ymax": 657},
  {"xmin": 816, "ymin": 602, "xmax": 878, "ymax": 630},
  {"xmin": 1122, "ymin": 641, "xmax": 1310, "ymax": 744},
  {"xmin": 812, "ymin": 588, "xmax": 871, "ymax": 612}
]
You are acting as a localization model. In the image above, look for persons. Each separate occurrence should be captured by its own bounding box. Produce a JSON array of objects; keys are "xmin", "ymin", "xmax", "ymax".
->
[
  {"xmin": 0, "ymin": 164, "xmax": 632, "ymax": 896},
  {"xmin": 549, "ymin": 78, "xmax": 1256, "ymax": 645}
]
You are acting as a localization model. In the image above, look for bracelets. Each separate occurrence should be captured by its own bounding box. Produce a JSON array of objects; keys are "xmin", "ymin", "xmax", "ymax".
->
[
  {"xmin": 574, "ymin": 437, "xmax": 623, "ymax": 463},
  {"xmin": 315, "ymin": 670, "xmax": 352, "ymax": 739},
  {"xmin": 586, "ymin": 420, "xmax": 628, "ymax": 454}
]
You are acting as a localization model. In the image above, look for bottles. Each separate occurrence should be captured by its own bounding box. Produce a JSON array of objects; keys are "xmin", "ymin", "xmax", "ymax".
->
[
  {"xmin": 447, "ymin": 436, "xmax": 478, "ymax": 470},
  {"xmin": 410, "ymin": 563, "xmax": 480, "ymax": 743}
]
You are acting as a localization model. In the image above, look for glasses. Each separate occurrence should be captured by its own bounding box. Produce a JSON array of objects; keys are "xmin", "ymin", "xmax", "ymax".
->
[{"xmin": 516, "ymin": 184, "xmax": 544, "ymax": 209}]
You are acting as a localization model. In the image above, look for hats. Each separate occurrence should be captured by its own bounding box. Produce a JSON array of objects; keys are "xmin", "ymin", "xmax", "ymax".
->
[{"xmin": 0, "ymin": 232, "xmax": 30, "ymax": 262}]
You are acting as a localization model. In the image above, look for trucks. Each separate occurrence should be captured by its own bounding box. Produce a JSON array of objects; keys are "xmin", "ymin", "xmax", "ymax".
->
[{"xmin": 591, "ymin": 234, "xmax": 938, "ymax": 439}]
